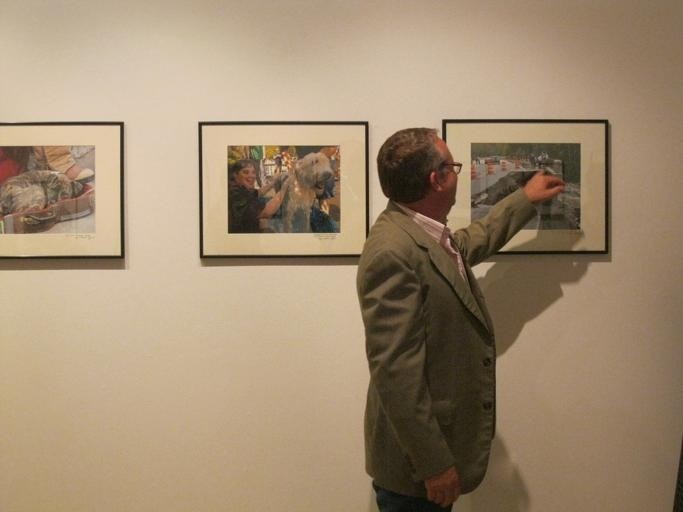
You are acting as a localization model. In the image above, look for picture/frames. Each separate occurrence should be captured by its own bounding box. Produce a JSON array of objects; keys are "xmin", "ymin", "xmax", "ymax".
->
[
  {"xmin": 198, "ymin": 120, "xmax": 369, "ymax": 259},
  {"xmin": 442, "ymin": 119, "xmax": 608, "ymax": 256},
  {"xmin": 0, "ymin": 122, "xmax": 124, "ymax": 260}
]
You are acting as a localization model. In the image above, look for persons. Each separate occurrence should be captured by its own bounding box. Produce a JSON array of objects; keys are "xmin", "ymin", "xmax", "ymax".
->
[
  {"xmin": 228, "ymin": 146, "xmax": 295, "ymax": 234},
  {"xmin": 30, "ymin": 146, "xmax": 95, "ymax": 185},
  {"xmin": 356, "ymin": 126, "xmax": 567, "ymax": 511}
]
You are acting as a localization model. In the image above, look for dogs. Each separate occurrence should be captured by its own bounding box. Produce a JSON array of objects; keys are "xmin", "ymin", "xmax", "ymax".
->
[
  {"xmin": 0, "ymin": 170, "xmax": 83, "ymax": 216},
  {"xmin": 261, "ymin": 152, "xmax": 336, "ymax": 235}
]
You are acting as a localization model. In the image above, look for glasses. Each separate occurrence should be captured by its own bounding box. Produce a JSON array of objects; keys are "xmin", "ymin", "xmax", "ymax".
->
[{"xmin": 438, "ymin": 161, "xmax": 462, "ymax": 175}]
